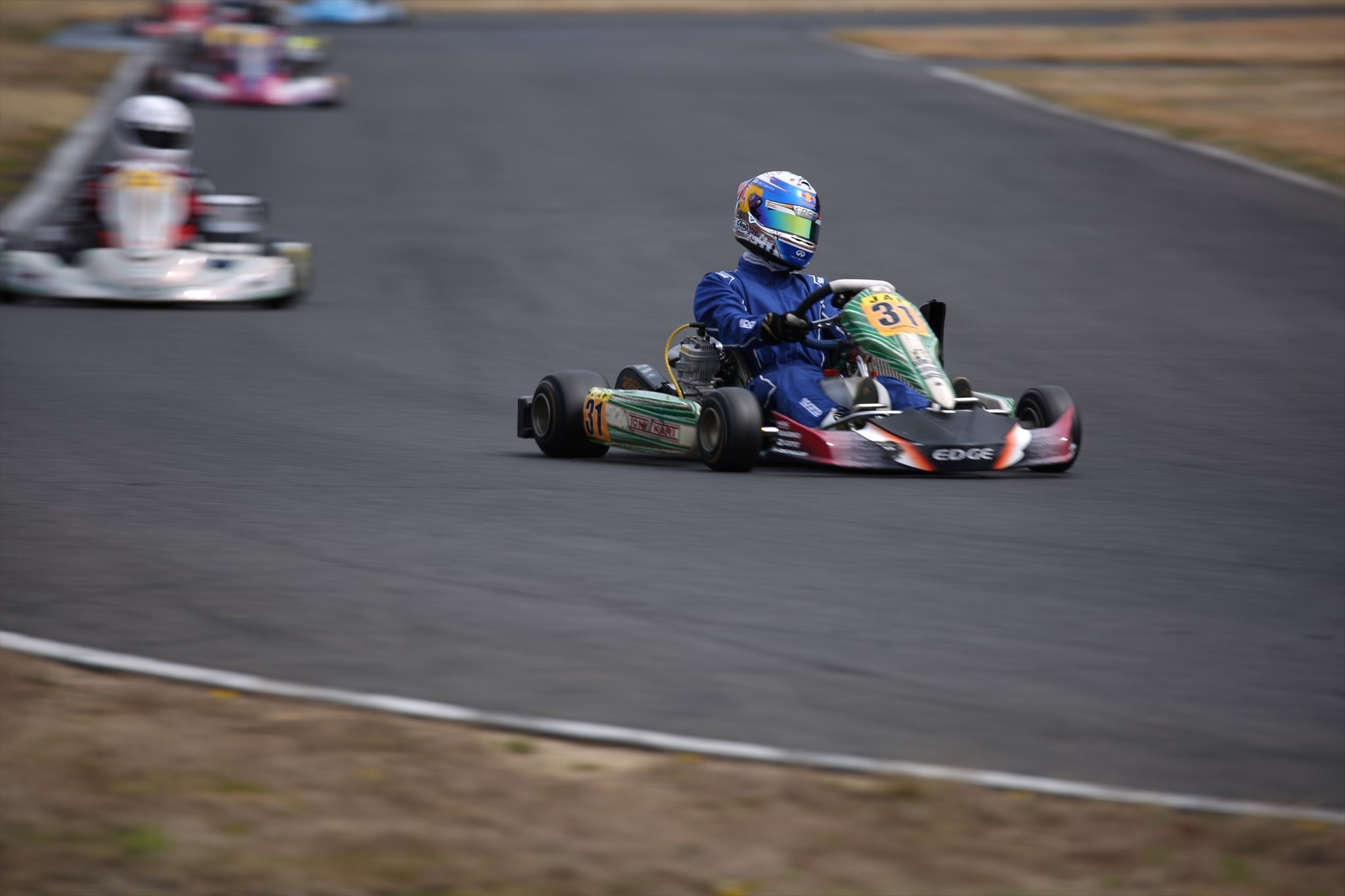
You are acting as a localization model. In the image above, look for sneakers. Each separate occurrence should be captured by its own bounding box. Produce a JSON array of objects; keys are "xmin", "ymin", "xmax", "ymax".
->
[
  {"xmin": 833, "ymin": 377, "xmax": 891, "ymax": 432},
  {"xmin": 940, "ymin": 376, "xmax": 975, "ymax": 411}
]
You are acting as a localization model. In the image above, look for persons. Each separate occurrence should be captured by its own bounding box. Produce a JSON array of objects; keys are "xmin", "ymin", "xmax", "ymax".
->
[
  {"xmin": 183, "ymin": 0, "xmax": 335, "ymax": 78},
  {"xmin": 51, "ymin": 91, "xmax": 260, "ymax": 269},
  {"xmin": 694, "ymin": 168, "xmax": 975, "ymax": 436}
]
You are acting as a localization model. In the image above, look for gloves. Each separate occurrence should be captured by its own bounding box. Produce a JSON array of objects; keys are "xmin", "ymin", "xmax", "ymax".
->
[{"xmin": 760, "ymin": 312, "xmax": 813, "ymax": 345}]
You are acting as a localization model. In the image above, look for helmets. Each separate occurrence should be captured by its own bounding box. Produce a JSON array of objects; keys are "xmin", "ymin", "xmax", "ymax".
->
[
  {"xmin": 733, "ymin": 171, "xmax": 821, "ymax": 269},
  {"xmin": 112, "ymin": 96, "xmax": 195, "ymax": 172}
]
